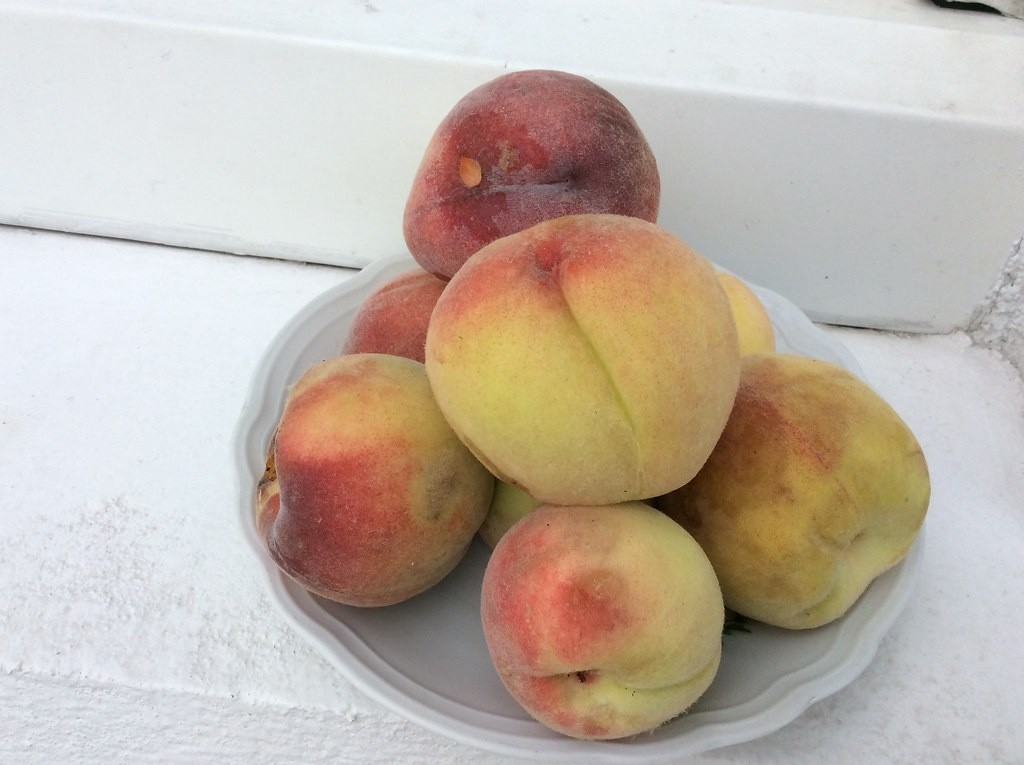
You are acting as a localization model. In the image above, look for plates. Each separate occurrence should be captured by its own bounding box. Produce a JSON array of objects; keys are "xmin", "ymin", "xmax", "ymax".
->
[{"xmin": 232, "ymin": 255, "xmax": 925, "ymax": 765}]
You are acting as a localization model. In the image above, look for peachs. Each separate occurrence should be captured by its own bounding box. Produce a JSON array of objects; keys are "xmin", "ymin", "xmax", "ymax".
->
[{"xmin": 256, "ymin": 70, "xmax": 930, "ymax": 740}]
